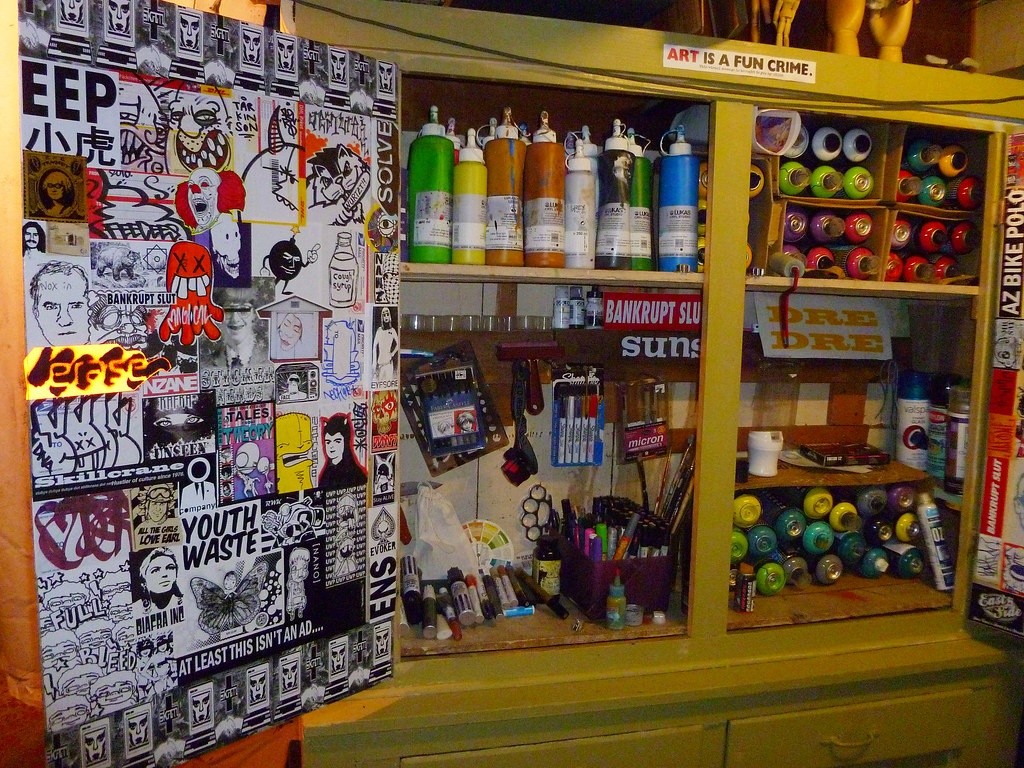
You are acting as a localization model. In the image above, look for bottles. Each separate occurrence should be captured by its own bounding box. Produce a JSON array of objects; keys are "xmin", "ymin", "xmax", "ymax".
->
[
  {"xmin": 595, "ymin": 119, "xmax": 636, "ymax": 270},
  {"xmin": 567, "ymin": 140, "xmax": 598, "ymax": 268},
  {"xmin": 526, "ymin": 112, "xmax": 566, "ymax": 269},
  {"xmin": 484, "ymin": 106, "xmax": 527, "ymax": 266},
  {"xmin": 532, "ymin": 536, "xmax": 561, "ymax": 604},
  {"xmin": 728, "ymin": 486, "xmax": 954, "ymax": 592},
  {"xmin": 627, "ymin": 128, "xmax": 652, "ymax": 270},
  {"xmin": 885, "ymin": 141, "xmax": 982, "ymax": 282},
  {"xmin": 658, "ymin": 125, "xmax": 700, "ymax": 272},
  {"xmin": 453, "ymin": 128, "xmax": 487, "ymax": 265},
  {"xmin": 408, "ymin": 105, "xmax": 455, "ymax": 265},
  {"xmin": 782, "ymin": 126, "xmax": 882, "ymax": 277}
]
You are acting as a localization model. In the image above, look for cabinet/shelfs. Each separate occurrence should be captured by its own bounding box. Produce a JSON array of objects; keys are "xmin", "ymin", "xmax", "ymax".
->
[{"xmin": 280, "ymin": 0, "xmax": 1024, "ymax": 768}]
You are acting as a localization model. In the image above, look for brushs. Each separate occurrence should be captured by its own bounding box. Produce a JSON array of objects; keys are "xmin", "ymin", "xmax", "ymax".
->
[
  {"xmin": 494, "ymin": 340, "xmax": 556, "ymax": 414},
  {"xmin": 501, "ymin": 358, "xmax": 538, "ymax": 489}
]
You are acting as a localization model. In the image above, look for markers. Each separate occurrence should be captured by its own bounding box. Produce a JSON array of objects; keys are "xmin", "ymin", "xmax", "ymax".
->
[
  {"xmin": 557, "ymin": 393, "xmax": 597, "ymax": 465},
  {"xmin": 422, "ymin": 583, "xmax": 437, "ymax": 638},
  {"xmin": 439, "ymin": 586, "xmax": 463, "ymax": 641},
  {"xmin": 556, "ymin": 493, "xmax": 672, "ymax": 559},
  {"xmin": 466, "ymin": 566, "xmax": 569, "ymax": 622},
  {"xmin": 448, "ymin": 567, "xmax": 475, "ymax": 627},
  {"xmin": 400, "ymin": 554, "xmax": 425, "ymax": 641}
]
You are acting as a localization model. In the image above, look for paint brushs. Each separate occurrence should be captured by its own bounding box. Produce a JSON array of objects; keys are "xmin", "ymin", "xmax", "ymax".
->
[
  {"xmin": 660, "ymin": 432, "xmax": 697, "ymax": 538},
  {"xmin": 637, "ymin": 451, "xmax": 650, "ymax": 511},
  {"xmin": 654, "ymin": 429, "xmax": 673, "ymax": 514}
]
